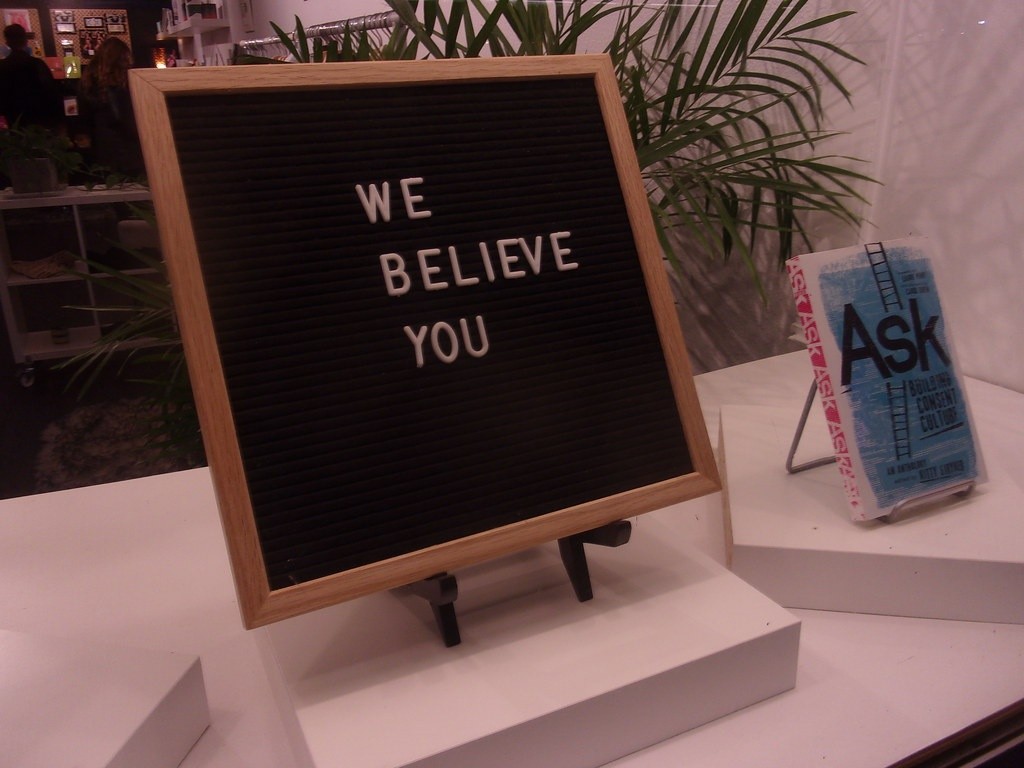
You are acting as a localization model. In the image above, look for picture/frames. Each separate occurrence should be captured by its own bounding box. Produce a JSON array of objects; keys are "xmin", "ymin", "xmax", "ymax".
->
[
  {"xmin": 56, "ymin": 22, "xmax": 77, "ymax": 34},
  {"xmin": 79, "ymin": 28, "xmax": 106, "ymax": 59},
  {"xmin": 130, "ymin": 53, "xmax": 723, "ymax": 632},
  {"xmin": 106, "ymin": 23, "xmax": 127, "ymax": 34},
  {"xmin": 84, "ymin": 16, "xmax": 104, "ymax": 28}
]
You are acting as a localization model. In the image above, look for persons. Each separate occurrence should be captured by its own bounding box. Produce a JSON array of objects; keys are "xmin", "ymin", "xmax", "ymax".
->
[
  {"xmin": 0, "ymin": 23, "xmax": 63, "ymax": 131},
  {"xmin": 66, "ymin": 36, "xmax": 147, "ymax": 270}
]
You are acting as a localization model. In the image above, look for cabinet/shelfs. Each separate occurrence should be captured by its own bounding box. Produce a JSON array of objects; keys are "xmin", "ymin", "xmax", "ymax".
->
[{"xmin": 0, "ymin": 183, "xmax": 182, "ymax": 363}]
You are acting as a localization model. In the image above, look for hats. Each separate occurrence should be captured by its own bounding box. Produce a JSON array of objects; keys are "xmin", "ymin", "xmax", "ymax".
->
[{"xmin": 3, "ymin": 24, "xmax": 34, "ymax": 46}]
